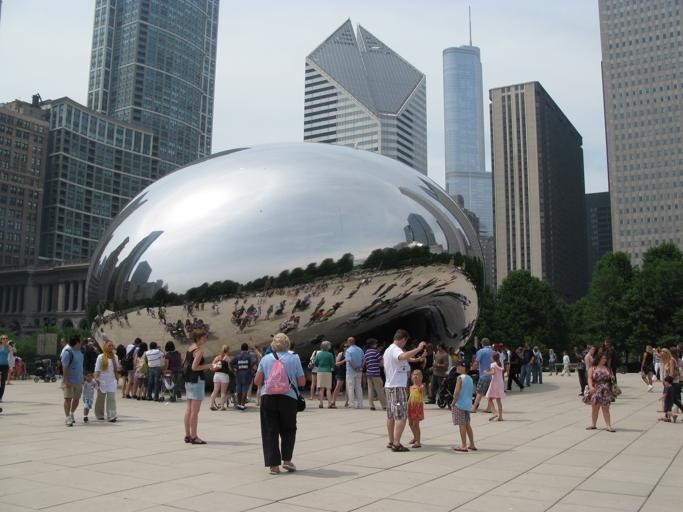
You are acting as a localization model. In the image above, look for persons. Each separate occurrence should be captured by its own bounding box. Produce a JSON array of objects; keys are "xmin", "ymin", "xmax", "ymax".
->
[
  {"xmin": 93, "ymin": 262, "xmax": 481, "ymax": 352},
  {"xmin": 447, "ymin": 358, "xmax": 478, "ymax": 452},
  {"xmin": 252, "ymin": 331, "xmax": 306, "ymax": 477},
  {"xmin": 0, "ymin": 329, "xmax": 682, "ymax": 431},
  {"xmin": 183, "ymin": 331, "xmax": 222, "ymax": 444},
  {"xmin": 382, "ymin": 330, "xmax": 429, "ymax": 452}
]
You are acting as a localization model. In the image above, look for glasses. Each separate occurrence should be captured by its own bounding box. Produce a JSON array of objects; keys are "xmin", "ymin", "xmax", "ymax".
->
[{"xmin": 344, "ymin": 345, "xmax": 349, "ymax": 348}]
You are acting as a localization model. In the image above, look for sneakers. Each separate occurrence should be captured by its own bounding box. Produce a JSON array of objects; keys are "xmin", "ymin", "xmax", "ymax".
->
[
  {"xmin": 672, "ymin": 415, "xmax": 678, "ymax": 423},
  {"xmin": 65, "ymin": 413, "xmax": 117, "ymax": 426},
  {"xmin": 122, "ymin": 390, "xmax": 181, "ymax": 402}
]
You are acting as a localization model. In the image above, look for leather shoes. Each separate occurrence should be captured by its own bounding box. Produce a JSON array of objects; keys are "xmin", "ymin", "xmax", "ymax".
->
[{"xmin": 425, "ymin": 400, "xmax": 436, "ymax": 404}]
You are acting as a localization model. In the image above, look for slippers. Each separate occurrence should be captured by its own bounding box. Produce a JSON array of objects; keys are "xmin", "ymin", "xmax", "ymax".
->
[
  {"xmin": 586, "ymin": 425, "xmax": 596, "ymax": 429},
  {"xmin": 283, "ymin": 462, "xmax": 296, "ymax": 471},
  {"xmin": 412, "ymin": 443, "xmax": 421, "ymax": 448},
  {"xmin": 606, "ymin": 428, "xmax": 616, "ymax": 432},
  {"xmin": 409, "ymin": 439, "xmax": 415, "ymax": 443},
  {"xmin": 269, "ymin": 468, "xmax": 280, "ymax": 474},
  {"xmin": 210, "ymin": 404, "xmax": 247, "ymax": 410},
  {"xmin": 454, "ymin": 415, "xmax": 503, "ymax": 452}
]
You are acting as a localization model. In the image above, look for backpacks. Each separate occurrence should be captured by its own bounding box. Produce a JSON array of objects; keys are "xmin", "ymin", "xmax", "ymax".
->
[
  {"xmin": 533, "ymin": 352, "xmax": 540, "ymax": 365},
  {"xmin": 121, "ymin": 346, "xmax": 136, "ymax": 370},
  {"xmin": 57, "ymin": 348, "xmax": 74, "ymax": 375},
  {"xmin": 263, "ymin": 352, "xmax": 297, "ymax": 395}
]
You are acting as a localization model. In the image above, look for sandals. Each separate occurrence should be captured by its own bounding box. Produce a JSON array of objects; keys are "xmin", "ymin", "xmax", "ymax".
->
[
  {"xmin": 184, "ymin": 436, "xmax": 191, "ymax": 442},
  {"xmin": 190, "ymin": 436, "xmax": 206, "ymax": 444},
  {"xmin": 387, "ymin": 442, "xmax": 393, "ymax": 448},
  {"xmin": 392, "ymin": 443, "xmax": 409, "ymax": 452}
]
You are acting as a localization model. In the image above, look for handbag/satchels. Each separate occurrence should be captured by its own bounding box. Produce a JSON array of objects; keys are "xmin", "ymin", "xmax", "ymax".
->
[
  {"xmin": 210, "ymin": 361, "xmax": 222, "ymax": 372},
  {"xmin": 139, "ymin": 361, "xmax": 148, "ymax": 374},
  {"xmin": 297, "ymin": 395, "xmax": 305, "ymax": 411},
  {"xmin": 308, "ymin": 359, "xmax": 315, "ymax": 369},
  {"xmin": 182, "ymin": 360, "xmax": 192, "ymax": 382},
  {"xmin": 611, "ymin": 384, "xmax": 622, "ymax": 397}
]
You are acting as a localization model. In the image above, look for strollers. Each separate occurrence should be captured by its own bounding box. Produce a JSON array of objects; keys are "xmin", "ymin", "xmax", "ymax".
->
[
  {"xmin": 31, "ymin": 359, "xmax": 56, "ymax": 383},
  {"xmin": 435, "ymin": 366, "xmax": 479, "ymax": 409},
  {"xmin": 158, "ymin": 371, "xmax": 176, "ymax": 403}
]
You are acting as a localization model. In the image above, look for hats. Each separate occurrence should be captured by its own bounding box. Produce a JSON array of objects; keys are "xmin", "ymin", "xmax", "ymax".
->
[
  {"xmin": 480, "ymin": 338, "xmax": 489, "ymax": 343},
  {"xmin": 665, "ymin": 376, "xmax": 673, "ymax": 384}
]
